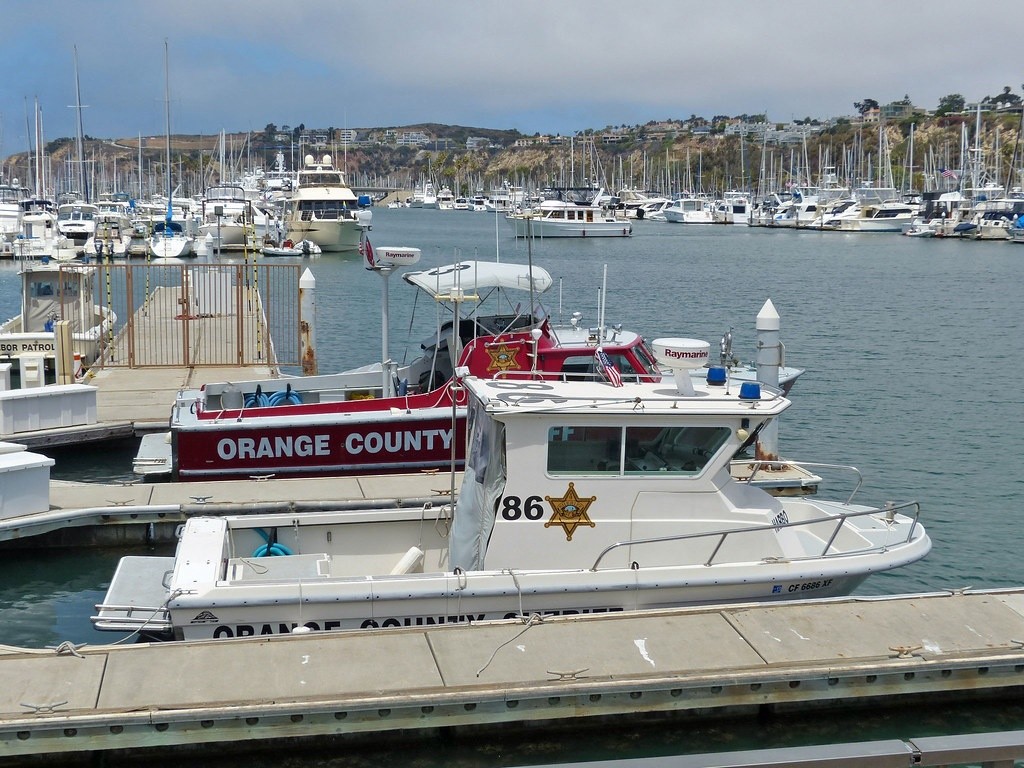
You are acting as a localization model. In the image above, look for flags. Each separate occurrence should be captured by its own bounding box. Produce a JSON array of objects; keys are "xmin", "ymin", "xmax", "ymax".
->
[
  {"xmin": 358, "ymin": 231, "xmax": 375, "ymax": 268},
  {"xmin": 598, "ymin": 351, "xmax": 623, "ymax": 388}
]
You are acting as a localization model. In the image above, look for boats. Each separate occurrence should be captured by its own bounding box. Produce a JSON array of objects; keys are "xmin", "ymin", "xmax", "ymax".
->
[
  {"xmin": 387, "ymin": 103, "xmax": 1024, "ymax": 242},
  {"xmin": 131, "ymin": 260, "xmax": 807, "ymax": 480},
  {"xmin": 93, "ymin": 376, "xmax": 935, "ymax": 639},
  {"xmin": 0, "ymin": 261, "xmax": 120, "ymax": 372},
  {"xmin": 0, "ymin": 36, "xmax": 374, "ymax": 262}
]
